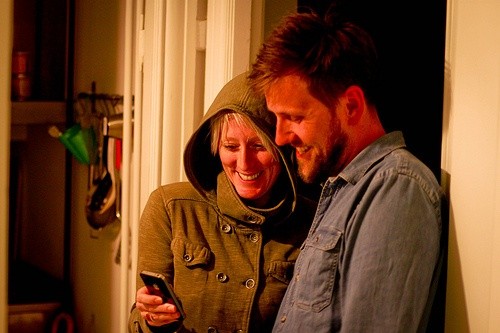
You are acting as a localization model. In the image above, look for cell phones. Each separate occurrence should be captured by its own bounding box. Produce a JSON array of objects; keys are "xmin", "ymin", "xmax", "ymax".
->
[{"xmin": 140, "ymin": 270, "xmax": 187, "ymax": 321}]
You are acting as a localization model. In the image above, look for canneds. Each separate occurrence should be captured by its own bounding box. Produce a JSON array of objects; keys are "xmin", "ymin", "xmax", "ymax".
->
[{"xmin": 11, "ymin": 50, "xmax": 30, "ymax": 101}]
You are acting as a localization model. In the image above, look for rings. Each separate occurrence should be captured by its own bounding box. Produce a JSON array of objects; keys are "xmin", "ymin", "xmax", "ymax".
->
[{"xmin": 147, "ymin": 312, "xmax": 155, "ymax": 322}]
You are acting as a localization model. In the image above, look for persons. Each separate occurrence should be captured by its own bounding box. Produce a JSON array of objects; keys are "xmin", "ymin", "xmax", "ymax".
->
[
  {"xmin": 128, "ymin": 71, "xmax": 321, "ymax": 333},
  {"xmin": 246, "ymin": 12, "xmax": 443, "ymax": 333}
]
